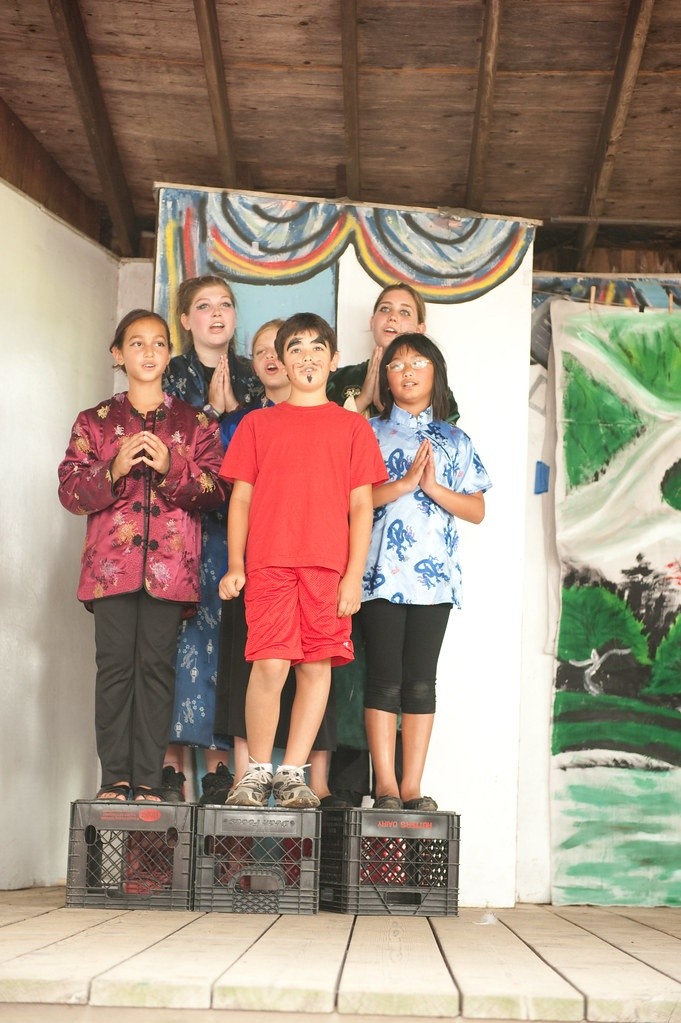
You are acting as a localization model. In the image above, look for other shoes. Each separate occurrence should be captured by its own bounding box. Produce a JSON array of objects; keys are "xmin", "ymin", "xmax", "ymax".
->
[
  {"xmin": 317, "ymin": 795, "xmax": 351, "ymax": 807},
  {"xmin": 403, "ymin": 796, "xmax": 439, "ymax": 812},
  {"xmin": 373, "ymin": 794, "xmax": 403, "ymax": 809}
]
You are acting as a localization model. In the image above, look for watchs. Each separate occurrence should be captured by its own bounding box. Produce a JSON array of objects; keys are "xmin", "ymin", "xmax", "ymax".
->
[{"xmin": 204, "ymin": 403, "xmax": 221, "ymax": 419}]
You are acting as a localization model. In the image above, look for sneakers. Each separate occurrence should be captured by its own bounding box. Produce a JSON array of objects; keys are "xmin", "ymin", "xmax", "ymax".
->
[
  {"xmin": 225, "ymin": 755, "xmax": 273, "ymax": 807},
  {"xmin": 271, "ymin": 763, "xmax": 321, "ymax": 808},
  {"xmin": 161, "ymin": 765, "xmax": 186, "ymax": 802},
  {"xmin": 200, "ymin": 761, "xmax": 234, "ymax": 804}
]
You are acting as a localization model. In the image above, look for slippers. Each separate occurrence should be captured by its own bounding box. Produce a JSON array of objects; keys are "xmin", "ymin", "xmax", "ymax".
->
[
  {"xmin": 133, "ymin": 787, "xmax": 165, "ymax": 802},
  {"xmin": 97, "ymin": 785, "xmax": 129, "ymax": 800}
]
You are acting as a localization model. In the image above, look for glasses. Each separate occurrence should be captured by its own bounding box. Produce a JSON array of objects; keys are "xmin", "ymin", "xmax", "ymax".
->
[{"xmin": 386, "ymin": 358, "xmax": 431, "ymax": 372}]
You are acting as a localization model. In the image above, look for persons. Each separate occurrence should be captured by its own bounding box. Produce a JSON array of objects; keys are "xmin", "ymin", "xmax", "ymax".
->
[
  {"xmin": 160, "ymin": 275, "xmax": 267, "ymax": 804},
  {"xmin": 58, "ymin": 308, "xmax": 234, "ymax": 803},
  {"xmin": 359, "ymin": 333, "xmax": 494, "ymax": 810},
  {"xmin": 329, "ymin": 280, "xmax": 463, "ymax": 805},
  {"xmin": 217, "ymin": 313, "xmax": 388, "ymax": 808},
  {"xmin": 220, "ymin": 318, "xmax": 351, "ymax": 808}
]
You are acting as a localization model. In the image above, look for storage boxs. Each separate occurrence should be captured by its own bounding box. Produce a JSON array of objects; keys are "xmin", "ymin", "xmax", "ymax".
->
[
  {"xmin": 317, "ymin": 805, "xmax": 462, "ymax": 918},
  {"xmin": 193, "ymin": 803, "xmax": 323, "ymax": 914},
  {"xmin": 64, "ymin": 798, "xmax": 196, "ymax": 913}
]
